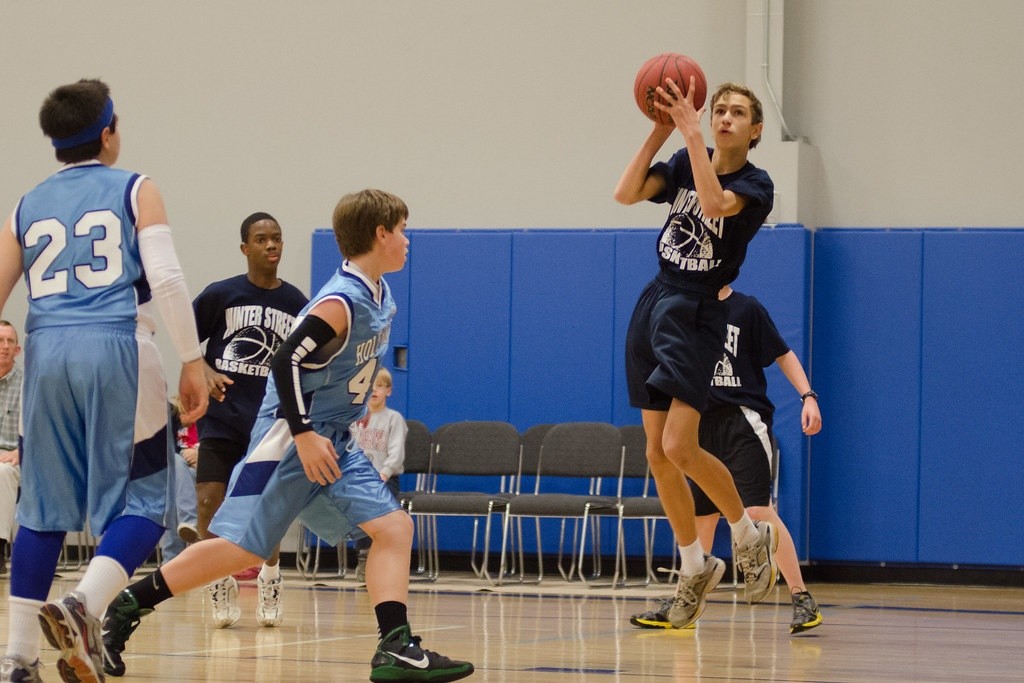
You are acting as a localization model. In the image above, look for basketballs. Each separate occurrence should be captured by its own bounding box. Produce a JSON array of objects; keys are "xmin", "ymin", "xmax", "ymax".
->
[{"xmin": 635, "ymin": 53, "xmax": 707, "ymax": 127}]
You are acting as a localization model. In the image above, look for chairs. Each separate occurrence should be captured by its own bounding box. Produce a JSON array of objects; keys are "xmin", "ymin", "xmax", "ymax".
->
[{"xmin": 294, "ymin": 420, "xmax": 782, "ymax": 590}]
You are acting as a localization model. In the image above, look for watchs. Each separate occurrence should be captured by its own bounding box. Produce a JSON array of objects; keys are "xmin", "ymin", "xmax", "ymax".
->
[{"xmin": 800, "ymin": 391, "xmax": 819, "ymax": 403}]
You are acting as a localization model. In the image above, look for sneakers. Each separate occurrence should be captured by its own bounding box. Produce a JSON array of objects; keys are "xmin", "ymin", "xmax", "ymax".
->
[
  {"xmin": 370, "ymin": 623, "xmax": 475, "ymax": 683},
  {"xmin": 355, "ymin": 549, "xmax": 369, "ymax": 583},
  {"xmin": 630, "ymin": 597, "xmax": 696, "ymax": 629},
  {"xmin": 668, "ymin": 551, "xmax": 727, "ymax": 630},
  {"xmin": 257, "ymin": 571, "xmax": 283, "ymax": 627},
  {"xmin": 0, "ymin": 653, "xmax": 44, "ymax": 683},
  {"xmin": 103, "ymin": 588, "xmax": 155, "ymax": 676},
  {"xmin": 788, "ymin": 592, "xmax": 823, "ymax": 635},
  {"xmin": 736, "ymin": 520, "xmax": 779, "ymax": 605},
  {"xmin": 37, "ymin": 590, "xmax": 106, "ymax": 683},
  {"xmin": 208, "ymin": 575, "xmax": 242, "ymax": 630}
]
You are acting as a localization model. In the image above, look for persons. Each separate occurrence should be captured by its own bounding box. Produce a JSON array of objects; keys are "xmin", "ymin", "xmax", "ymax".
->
[
  {"xmin": 191, "ymin": 211, "xmax": 312, "ymax": 628},
  {"xmin": 161, "ymin": 398, "xmax": 201, "ymax": 563},
  {"xmin": 0, "ymin": 319, "xmax": 25, "ymax": 574},
  {"xmin": 613, "ymin": 74, "xmax": 778, "ymax": 629},
  {"xmin": 105, "ymin": 192, "xmax": 477, "ymax": 683},
  {"xmin": 628, "ymin": 283, "xmax": 822, "ymax": 633},
  {"xmin": 0, "ymin": 75, "xmax": 210, "ymax": 683},
  {"xmin": 353, "ymin": 368, "xmax": 407, "ymax": 583}
]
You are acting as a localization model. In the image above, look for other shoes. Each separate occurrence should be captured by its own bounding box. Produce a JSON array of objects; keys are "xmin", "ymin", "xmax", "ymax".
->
[{"xmin": 177, "ymin": 523, "xmax": 203, "ymax": 544}]
